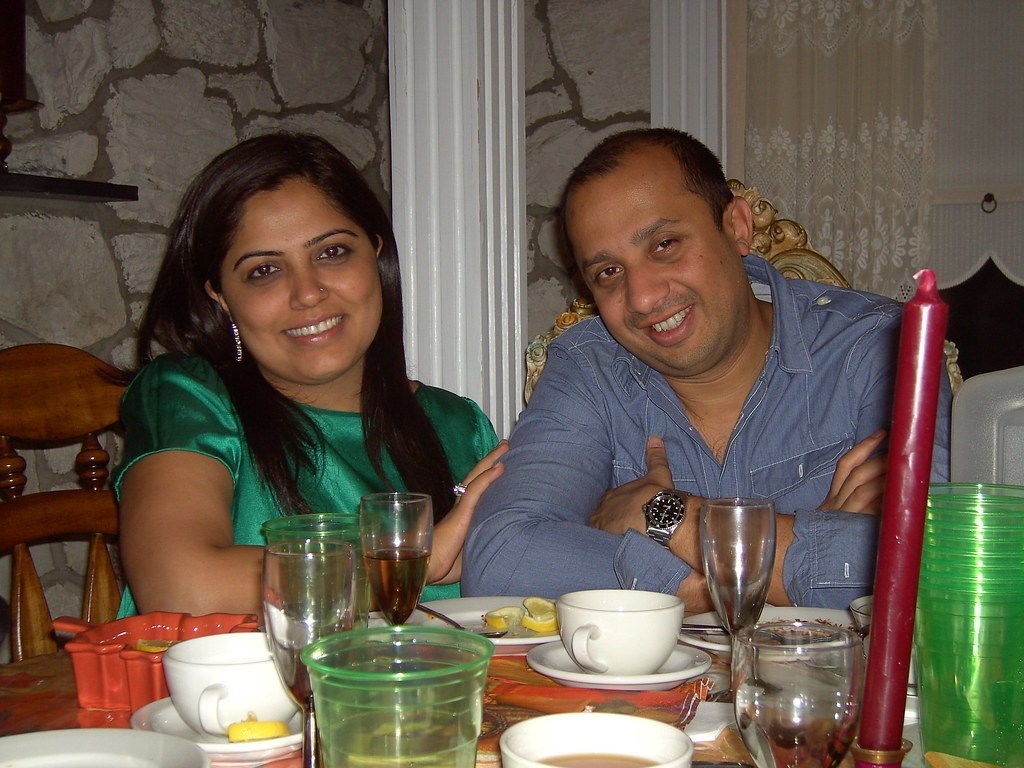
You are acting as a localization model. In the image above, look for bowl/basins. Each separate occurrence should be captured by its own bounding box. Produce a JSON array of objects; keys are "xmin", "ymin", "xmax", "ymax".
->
[{"xmin": 53, "ymin": 610, "xmax": 258, "ymax": 707}]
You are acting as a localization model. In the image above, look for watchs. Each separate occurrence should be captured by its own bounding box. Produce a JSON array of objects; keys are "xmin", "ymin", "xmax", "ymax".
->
[{"xmin": 642, "ymin": 488, "xmax": 692, "ymax": 545}]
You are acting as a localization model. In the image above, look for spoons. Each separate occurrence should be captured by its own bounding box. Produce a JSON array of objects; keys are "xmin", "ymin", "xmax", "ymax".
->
[{"xmin": 415, "ymin": 603, "xmax": 508, "ymax": 638}]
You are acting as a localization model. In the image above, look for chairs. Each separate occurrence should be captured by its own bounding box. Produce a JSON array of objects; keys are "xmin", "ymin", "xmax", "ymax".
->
[
  {"xmin": 519, "ymin": 175, "xmax": 964, "ymax": 433},
  {"xmin": 0, "ymin": 341, "xmax": 136, "ymax": 659}
]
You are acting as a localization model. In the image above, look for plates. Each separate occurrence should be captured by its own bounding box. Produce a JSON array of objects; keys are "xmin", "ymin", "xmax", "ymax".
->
[
  {"xmin": 678, "ymin": 607, "xmax": 852, "ymax": 662},
  {"xmin": 526, "ymin": 638, "xmax": 711, "ymax": 691},
  {"xmin": 129, "ymin": 696, "xmax": 304, "ymax": 768},
  {"xmin": 368, "ymin": 597, "xmax": 560, "ymax": 645},
  {"xmin": 0, "ymin": 728, "xmax": 209, "ymax": 768}
]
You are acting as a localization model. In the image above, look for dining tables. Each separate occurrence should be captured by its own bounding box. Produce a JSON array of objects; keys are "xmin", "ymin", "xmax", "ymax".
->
[{"xmin": 0, "ymin": 640, "xmax": 925, "ymax": 768}]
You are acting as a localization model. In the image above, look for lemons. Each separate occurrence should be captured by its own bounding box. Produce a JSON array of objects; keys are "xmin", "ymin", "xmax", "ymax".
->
[
  {"xmin": 485, "ymin": 606, "xmax": 523, "ymax": 631},
  {"xmin": 521, "ymin": 597, "xmax": 559, "ymax": 632},
  {"xmin": 228, "ymin": 720, "xmax": 291, "ymax": 743},
  {"xmin": 135, "ymin": 637, "xmax": 180, "ymax": 652}
]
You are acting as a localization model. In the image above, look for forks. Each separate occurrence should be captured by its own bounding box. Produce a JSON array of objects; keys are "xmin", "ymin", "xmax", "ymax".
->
[
  {"xmin": 857, "ymin": 625, "xmax": 870, "ymax": 641},
  {"xmin": 680, "ymin": 623, "xmax": 837, "ymax": 645}
]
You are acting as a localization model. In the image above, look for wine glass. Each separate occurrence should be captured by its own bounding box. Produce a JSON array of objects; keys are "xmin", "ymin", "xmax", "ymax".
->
[
  {"xmin": 361, "ymin": 493, "xmax": 433, "ymax": 673},
  {"xmin": 262, "ymin": 540, "xmax": 356, "ymax": 768},
  {"xmin": 698, "ymin": 498, "xmax": 776, "ymax": 692}
]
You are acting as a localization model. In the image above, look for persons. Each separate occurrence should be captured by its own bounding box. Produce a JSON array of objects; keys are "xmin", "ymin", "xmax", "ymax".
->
[
  {"xmin": 459, "ymin": 128, "xmax": 953, "ymax": 618},
  {"xmin": 110, "ymin": 130, "xmax": 509, "ymax": 630}
]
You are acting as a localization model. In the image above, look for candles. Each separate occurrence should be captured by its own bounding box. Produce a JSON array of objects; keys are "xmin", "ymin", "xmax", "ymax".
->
[{"xmin": 859, "ymin": 268, "xmax": 948, "ymax": 749}]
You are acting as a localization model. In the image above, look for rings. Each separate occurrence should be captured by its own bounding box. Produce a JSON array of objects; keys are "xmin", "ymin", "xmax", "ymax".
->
[{"xmin": 454, "ymin": 483, "xmax": 468, "ymax": 498}]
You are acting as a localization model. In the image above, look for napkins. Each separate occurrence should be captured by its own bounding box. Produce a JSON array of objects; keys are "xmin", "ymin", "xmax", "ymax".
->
[{"xmin": 684, "ymin": 700, "xmax": 734, "ymax": 742}]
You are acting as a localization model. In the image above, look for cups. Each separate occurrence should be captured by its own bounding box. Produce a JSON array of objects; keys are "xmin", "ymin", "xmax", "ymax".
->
[
  {"xmin": 261, "ymin": 513, "xmax": 367, "ymax": 628},
  {"xmin": 849, "ymin": 594, "xmax": 918, "ymax": 685},
  {"xmin": 161, "ymin": 633, "xmax": 300, "ymax": 743},
  {"xmin": 499, "ymin": 712, "xmax": 694, "ymax": 768},
  {"xmin": 300, "ymin": 626, "xmax": 495, "ymax": 768},
  {"xmin": 913, "ymin": 482, "xmax": 1024, "ymax": 768},
  {"xmin": 732, "ymin": 623, "xmax": 865, "ymax": 768},
  {"xmin": 557, "ymin": 589, "xmax": 684, "ymax": 675}
]
